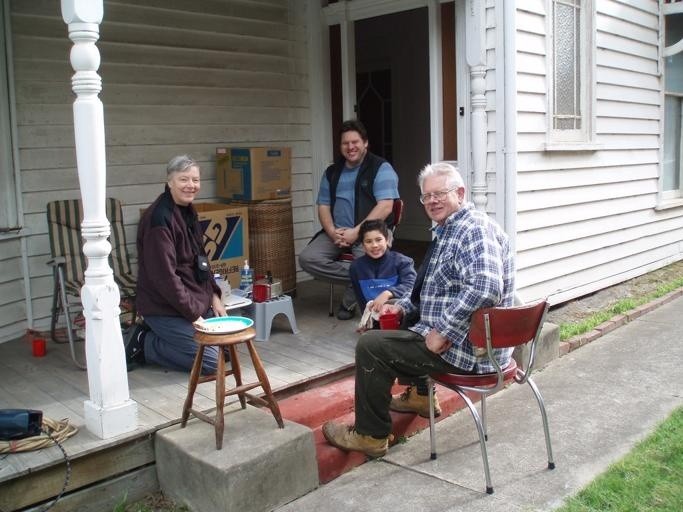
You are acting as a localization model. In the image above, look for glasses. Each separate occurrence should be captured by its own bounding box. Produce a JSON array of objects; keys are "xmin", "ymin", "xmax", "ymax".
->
[{"xmin": 420, "ymin": 187, "xmax": 459, "ymax": 205}]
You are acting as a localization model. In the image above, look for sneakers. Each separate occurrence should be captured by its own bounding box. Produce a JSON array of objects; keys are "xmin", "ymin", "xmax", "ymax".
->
[
  {"xmin": 337, "ymin": 304, "xmax": 355, "ymax": 320},
  {"xmin": 124, "ymin": 319, "xmax": 150, "ymax": 371},
  {"xmin": 388, "ymin": 385, "xmax": 442, "ymax": 418},
  {"xmin": 322, "ymin": 422, "xmax": 388, "ymax": 457}
]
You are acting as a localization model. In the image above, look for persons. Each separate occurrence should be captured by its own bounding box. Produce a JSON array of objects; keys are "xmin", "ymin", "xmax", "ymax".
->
[
  {"xmin": 319, "ymin": 159, "xmax": 517, "ymax": 460},
  {"xmin": 299, "ymin": 118, "xmax": 399, "ymax": 322},
  {"xmin": 347, "ymin": 218, "xmax": 418, "ymax": 334},
  {"xmin": 117, "ymin": 154, "xmax": 235, "ymax": 376}
]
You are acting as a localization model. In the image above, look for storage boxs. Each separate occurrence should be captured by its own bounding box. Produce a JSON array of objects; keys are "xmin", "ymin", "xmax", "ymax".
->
[
  {"xmin": 138, "ymin": 202, "xmax": 249, "ymax": 289},
  {"xmin": 215, "ymin": 147, "xmax": 291, "ymax": 201}
]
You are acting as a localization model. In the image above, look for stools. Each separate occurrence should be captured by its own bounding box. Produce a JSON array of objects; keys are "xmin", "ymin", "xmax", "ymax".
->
[
  {"xmin": 241, "ymin": 295, "xmax": 297, "ymax": 342},
  {"xmin": 180, "ymin": 327, "xmax": 284, "ymax": 449}
]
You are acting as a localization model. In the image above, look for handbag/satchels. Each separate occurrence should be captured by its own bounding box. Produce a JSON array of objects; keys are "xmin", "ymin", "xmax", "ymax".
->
[{"xmin": 195, "ymin": 255, "xmax": 210, "ymax": 283}]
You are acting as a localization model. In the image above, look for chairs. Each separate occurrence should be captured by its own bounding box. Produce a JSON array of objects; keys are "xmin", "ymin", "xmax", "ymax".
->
[
  {"xmin": 45, "ymin": 197, "xmax": 138, "ymax": 370},
  {"xmin": 328, "ymin": 199, "xmax": 403, "ymax": 317},
  {"xmin": 425, "ymin": 296, "xmax": 555, "ymax": 494}
]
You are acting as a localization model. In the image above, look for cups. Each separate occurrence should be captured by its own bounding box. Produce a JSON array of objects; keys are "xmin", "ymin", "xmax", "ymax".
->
[
  {"xmin": 32, "ymin": 339, "xmax": 46, "ymax": 357},
  {"xmin": 253, "ymin": 285, "xmax": 271, "ymax": 302},
  {"xmin": 380, "ymin": 313, "xmax": 401, "ymax": 330}
]
